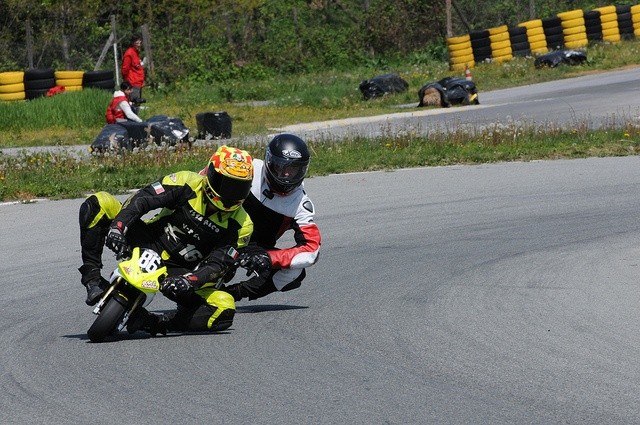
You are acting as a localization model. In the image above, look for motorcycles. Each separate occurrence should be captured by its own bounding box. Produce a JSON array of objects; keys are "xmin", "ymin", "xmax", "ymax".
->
[
  {"xmin": 214, "ymin": 252, "xmax": 260, "ymax": 290},
  {"xmin": 87, "ymin": 236, "xmax": 183, "ymax": 343}
]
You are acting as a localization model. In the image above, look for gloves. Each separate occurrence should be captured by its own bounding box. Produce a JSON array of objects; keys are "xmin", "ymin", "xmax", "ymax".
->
[
  {"xmin": 105, "ymin": 221, "xmax": 129, "ymax": 260},
  {"xmin": 241, "ymin": 250, "xmax": 273, "ymax": 277},
  {"xmin": 159, "ymin": 275, "xmax": 192, "ymax": 300}
]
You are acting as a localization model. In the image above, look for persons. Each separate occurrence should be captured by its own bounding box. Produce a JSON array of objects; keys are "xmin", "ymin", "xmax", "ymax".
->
[
  {"xmin": 105, "ymin": 81, "xmax": 143, "ymax": 125},
  {"xmin": 121, "ymin": 34, "xmax": 145, "ymax": 111},
  {"xmin": 77, "ymin": 146, "xmax": 255, "ymax": 335},
  {"xmin": 201, "ymin": 134, "xmax": 322, "ymax": 301}
]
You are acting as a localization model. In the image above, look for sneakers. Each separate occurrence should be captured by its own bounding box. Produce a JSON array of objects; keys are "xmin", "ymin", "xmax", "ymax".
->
[
  {"xmin": 80, "ymin": 266, "xmax": 109, "ymax": 306},
  {"xmin": 126, "ymin": 309, "xmax": 158, "ymax": 337}
]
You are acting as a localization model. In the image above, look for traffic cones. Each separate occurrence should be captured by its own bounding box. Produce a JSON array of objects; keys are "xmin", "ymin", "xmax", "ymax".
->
[{"xmin": 465, "ymin": 63, "xmax": 472, "ymax": 81}]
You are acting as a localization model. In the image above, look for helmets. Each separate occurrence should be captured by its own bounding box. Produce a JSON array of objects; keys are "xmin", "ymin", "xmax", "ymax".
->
[
  {"xmin": 202, "ymin": 145, "xmax": 254, "ymax": 212},
  {"xmin": 263, "ymin": 134, "xmax": 309, "ymax": 194}
]
